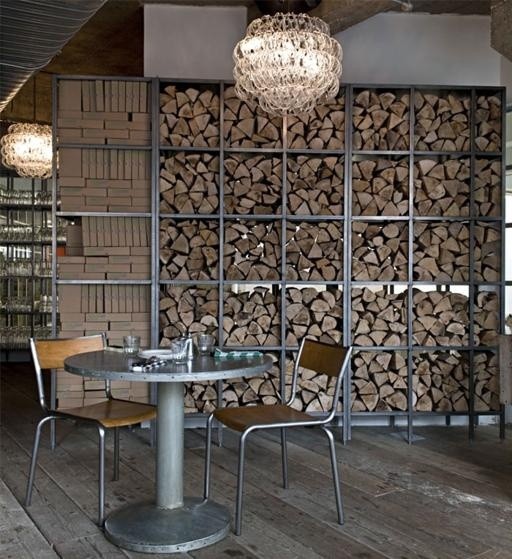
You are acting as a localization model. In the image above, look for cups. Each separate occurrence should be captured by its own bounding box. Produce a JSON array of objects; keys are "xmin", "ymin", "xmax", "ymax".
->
[
  {"xmin": 197, "ymin": 335, "xmax": 216, "ymax": 356},
  {"xmin": 172, "ymin": 341, "xmax": 189, "ymax": 365},
  {"xmin": 122, "ymin": 336, "xmax": 140, "ymax": 358}
]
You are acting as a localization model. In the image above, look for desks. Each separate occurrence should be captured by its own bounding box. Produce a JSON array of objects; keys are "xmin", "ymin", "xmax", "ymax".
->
[{"xmin": 63, "ymin": 348, "xmax": 273, "ymax": 554}]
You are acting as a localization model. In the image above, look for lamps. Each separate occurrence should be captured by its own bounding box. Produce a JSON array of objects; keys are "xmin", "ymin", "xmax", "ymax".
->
[
  {"xmin": 1, "ymin": 75, "xmax": 60, "ymax": 179},
  {"xmin": 232, "ymin": 12, "xmax": 343, "ymax": 118}
]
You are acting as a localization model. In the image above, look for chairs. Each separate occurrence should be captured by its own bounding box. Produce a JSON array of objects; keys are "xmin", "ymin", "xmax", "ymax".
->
[
  {"xmin": 203, "ymin": 336, "xmax": 352, "ymax": 536},
  {"xmin": 26, "ymin": 332, "xmax": 158, "ymax": 526}
]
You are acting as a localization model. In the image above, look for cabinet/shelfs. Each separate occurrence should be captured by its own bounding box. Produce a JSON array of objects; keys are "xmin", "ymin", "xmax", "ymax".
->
[
  {"xmin": 1, "ymin": 171, "xmax": 76, "ymax": 362},
  {"xmin": 52, "ymin": 75, "xmax": 506, "ymax": 446}
]
